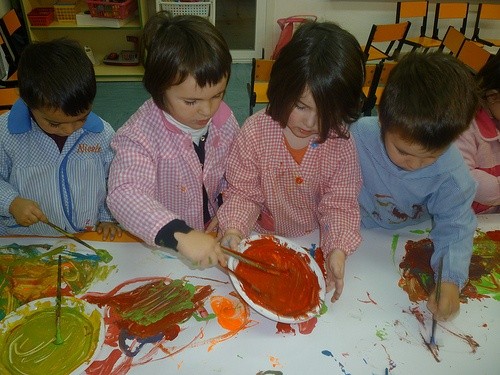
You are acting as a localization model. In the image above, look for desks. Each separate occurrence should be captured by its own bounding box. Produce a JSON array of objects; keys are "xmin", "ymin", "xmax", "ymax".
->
[{"xmin": 0, "ymin": 213, "xmax": 500, "ymax": 375}]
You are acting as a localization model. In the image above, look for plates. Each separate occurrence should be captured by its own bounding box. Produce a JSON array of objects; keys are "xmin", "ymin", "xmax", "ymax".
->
[
  {"xmin": 0, "ymin": 295, "xmax": 105, "ymax": 375},
  {"xmin": 227, "ymin": 233, "xmax": 325, "ymax": 323}
]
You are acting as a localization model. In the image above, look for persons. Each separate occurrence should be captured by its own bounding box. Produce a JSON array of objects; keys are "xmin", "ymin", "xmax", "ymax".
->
[
  {"xmin": 107, "ymin": 9, "xmax": 239, "ymax": 268},
  {"xmin": 217, "ymin": 20, "xmax": 368, "ymax": 303},
  {"xmin": 0, "ymin": 38, "xmax": 124, "ymax": 241},
  {"xmin": 454, "ymin": 48, "xmax": 500, "ymax": 215},
  {"xmin": 347, "ymin": 52, "xmax": 478, "ymax": 320}
]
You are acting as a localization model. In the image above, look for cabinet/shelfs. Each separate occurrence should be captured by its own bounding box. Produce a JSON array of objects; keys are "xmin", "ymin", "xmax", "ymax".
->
[{"xmin": 20, "ymin": 0, "xmax": 150, "ymax": 78}]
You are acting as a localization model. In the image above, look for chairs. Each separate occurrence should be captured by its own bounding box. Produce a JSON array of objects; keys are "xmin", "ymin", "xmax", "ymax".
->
[
  {"xmin": 246, "ymin": 0, "xmax": 500, "ymax": 117},
  {"xmin": 0, "ymin": 9, "xmax": 33, "ymax": 118}
]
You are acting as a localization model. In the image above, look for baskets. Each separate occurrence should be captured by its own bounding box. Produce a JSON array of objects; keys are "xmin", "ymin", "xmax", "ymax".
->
[
  {"xmin": 54, "ymin": 3, "xmax": 80, "ymax": 22},
  {"xmin": 87, "ymin": 0, "xmax": 138, "ymax": 18},
  {"xmin": 27, "ymin": 7, "xmax": 54, "ymax": 26},
  {"xmin": 160, "ymin": 2, "xmax": 211, "ymax": 17}
]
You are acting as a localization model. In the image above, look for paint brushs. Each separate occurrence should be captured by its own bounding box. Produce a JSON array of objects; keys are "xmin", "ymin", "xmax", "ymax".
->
[
  {"xmin": 431, "ymin": 256, "xmax": 443, "ymax": 348},
  {"xmin": 56, "ymin": 255, "xmax": 64, "ymax": 344},
  {"xmin": 44, "ymin": 221, "xmax": 112, "ymax": 263},
  {"xmin": 219, "ymin": 245, "xmax": 285, "ymax": 276},
  {"xmin": 218, "ymin": 261, "xmax": 264, "ymax": 296}
]
